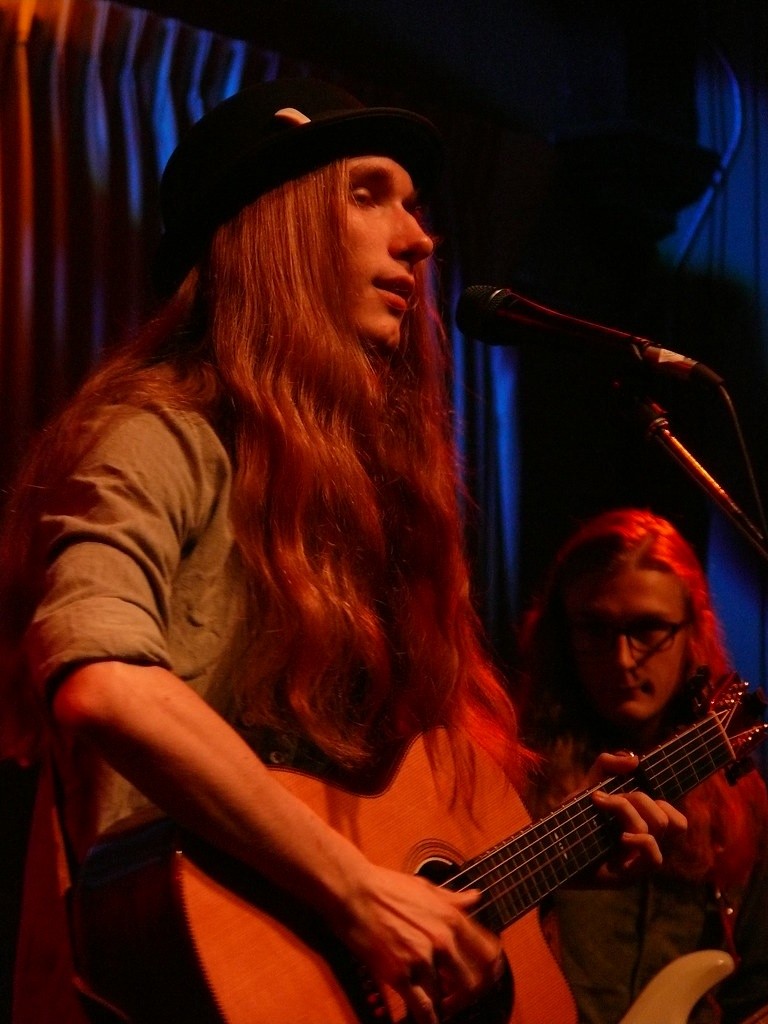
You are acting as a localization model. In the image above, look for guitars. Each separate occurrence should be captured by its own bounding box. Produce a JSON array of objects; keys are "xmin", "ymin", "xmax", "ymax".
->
[{"xmin": 70, "ymin": 664, "xmax": 763, "ymax": 1022}]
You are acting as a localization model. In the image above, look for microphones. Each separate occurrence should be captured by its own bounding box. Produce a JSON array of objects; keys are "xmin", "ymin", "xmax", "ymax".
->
[{"xmin": 453, "ymin": 281, "xmax": 721, "ymax": 399}]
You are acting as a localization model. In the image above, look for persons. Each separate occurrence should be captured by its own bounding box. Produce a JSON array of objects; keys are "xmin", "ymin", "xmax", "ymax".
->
[
  {"xmin": 0, "ymin": 73, "xmax": 688, "ymax": 1024},
  {"xmin": 507, "ymin": 510, "xmax": 768, "ymax": 1024}
]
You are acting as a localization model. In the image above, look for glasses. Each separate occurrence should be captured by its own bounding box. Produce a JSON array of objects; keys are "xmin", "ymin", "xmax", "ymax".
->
[{"xmin": 563, "ymin": 615, "xmax": 690, "ymax": 654}]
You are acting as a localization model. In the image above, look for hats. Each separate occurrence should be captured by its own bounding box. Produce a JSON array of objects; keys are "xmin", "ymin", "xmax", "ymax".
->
[{"xmin": 146, "ymin": 80, "xmax": 441, "ymax": 279}]
